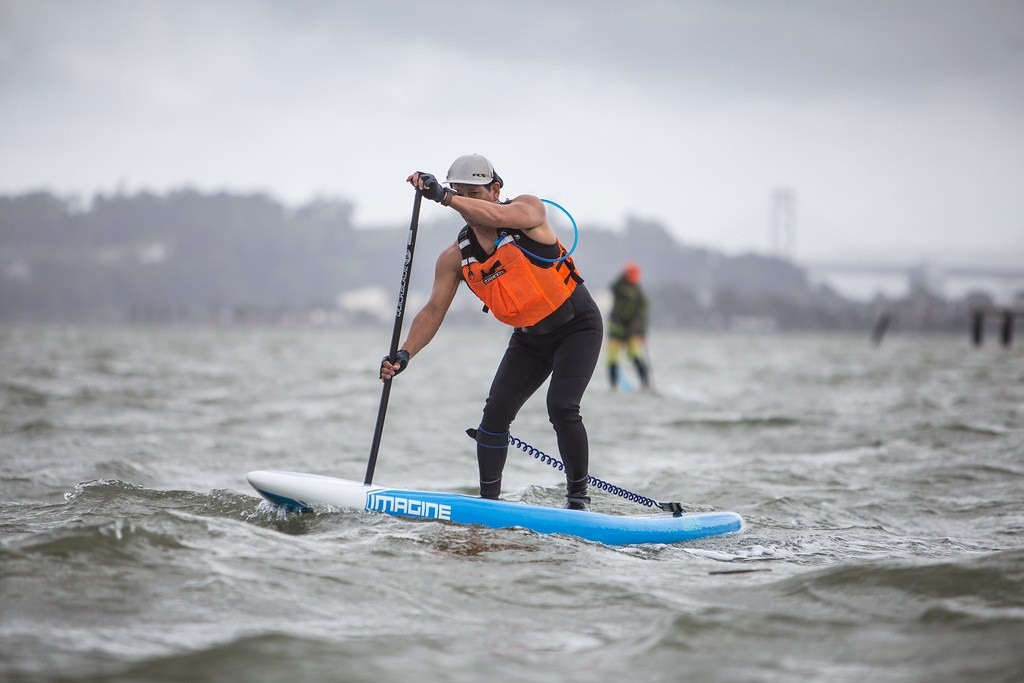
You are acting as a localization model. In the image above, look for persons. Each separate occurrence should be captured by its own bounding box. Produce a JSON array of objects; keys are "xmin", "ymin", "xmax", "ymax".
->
[
  {"xmin": 378, "ymin": 153, "xmax": 604, "ymax": 511},
  {"xmin": 608, "ymin": 264, "xmax": 651, "ymax": 393}
]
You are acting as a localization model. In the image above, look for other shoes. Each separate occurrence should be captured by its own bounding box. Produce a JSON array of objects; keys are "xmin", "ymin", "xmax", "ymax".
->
[{"xmin": 564, "ymin": 495, "xmax": 591, "ymax": 513}]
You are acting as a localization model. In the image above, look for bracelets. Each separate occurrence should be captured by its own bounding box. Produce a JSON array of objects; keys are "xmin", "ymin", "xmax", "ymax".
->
[{"xmin": 440, "ymin": 187, "xmax": 458, "ymax": 207}]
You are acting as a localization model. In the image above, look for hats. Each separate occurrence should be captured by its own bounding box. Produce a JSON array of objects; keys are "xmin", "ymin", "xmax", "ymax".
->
[
  {"xmin": 443, "ymin": 153, "xmax": 504, "ymax": 187},
  {"xmin": 626, "ymin": 263, "xmax": 641, "ymax": 283}
]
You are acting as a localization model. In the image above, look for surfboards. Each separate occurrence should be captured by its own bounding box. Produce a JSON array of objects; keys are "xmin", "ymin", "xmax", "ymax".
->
[{"xmin": 247, "ymin": 468, "xmax": 742, "ymax": 548}]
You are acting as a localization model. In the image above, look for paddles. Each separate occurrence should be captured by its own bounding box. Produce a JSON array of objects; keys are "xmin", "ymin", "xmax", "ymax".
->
[{"xmin": 362, "ymin": 175, "xmax": 424, "ymax": 487}]
